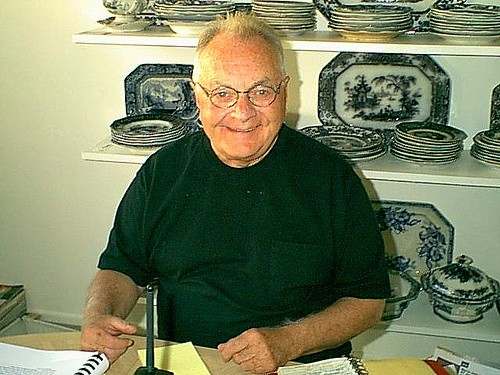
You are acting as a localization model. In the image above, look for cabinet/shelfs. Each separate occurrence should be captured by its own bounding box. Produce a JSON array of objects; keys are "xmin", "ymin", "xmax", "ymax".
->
[
  {"xmin": 138, "ymin": 286, "xmax": 500, "ymax": 372},
  {"xmin": 73, "ymin": 24, "xmax": 500, "ymax": 188}
]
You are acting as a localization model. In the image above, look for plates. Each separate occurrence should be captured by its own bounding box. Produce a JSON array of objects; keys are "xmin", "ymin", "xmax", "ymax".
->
[
  {"xmin": 318, "ymin": 52, "xmax": 452, "ymax": 126},
  {"xmin": 370, "ymin": 199, "xmax": 455, "ymax": 291},
  {"xmin": 97, "ymin": 18, "xmax": 152, "ymax": 32},
  {"xmin": 298, "ymin": 126, "xmax": 388, "ymax": 162},
  {"xmin": 151, "ymin": 0, "xmax": 500, "ymax": 46},
  {"xmin": 109, "ymin": 115, "xmax": 185, "ymax": 147},
  {"xmin": 470, "ymin": 130, "xmax": 500, "ymax": 166},
  {"xmin": 125, "ymin": 63, "xmax": 201, "ymax": 121},
  {"xmin": 390, "ymin": 121, "xmax": 468, "ymax": 166}
]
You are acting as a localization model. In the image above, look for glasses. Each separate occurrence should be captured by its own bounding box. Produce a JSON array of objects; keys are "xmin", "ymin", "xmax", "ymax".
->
[{"xmin": 189, "ymin": 75, "xmax": 291, "ymax": 108}]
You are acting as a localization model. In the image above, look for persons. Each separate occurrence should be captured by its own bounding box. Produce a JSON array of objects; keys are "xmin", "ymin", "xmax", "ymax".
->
[{"xmin": 79, "ymin": 11, "xmax": 392, "ymax": 375}]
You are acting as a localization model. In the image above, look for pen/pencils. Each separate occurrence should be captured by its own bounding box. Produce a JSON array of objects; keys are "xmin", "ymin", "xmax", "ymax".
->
[{"xmin": 145, "ymin": 284, "xmax": 155, "ymax": 374}]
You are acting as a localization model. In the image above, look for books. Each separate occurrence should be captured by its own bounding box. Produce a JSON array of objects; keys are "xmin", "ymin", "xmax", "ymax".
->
[
  {"xmin": 0, "ymin": 342, "xmax": 109, "ymax": 375},
  {"xmin": 0, "ymin": 284, "xmax": 27, "ymax": 330},
  {"xmin": 276, "ymin": 356, "xmax": 372, "ymax": 375}
]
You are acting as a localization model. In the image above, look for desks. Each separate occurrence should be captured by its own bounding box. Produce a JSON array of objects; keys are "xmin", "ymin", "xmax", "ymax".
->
[{"xmin": 1, "ymin": 316, "xmax": 304, "ymax": 375}]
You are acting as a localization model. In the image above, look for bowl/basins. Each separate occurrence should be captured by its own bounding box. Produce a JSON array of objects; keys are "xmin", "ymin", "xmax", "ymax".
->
[
  {"xmin": 381, "ymin": 269, "xmax": 420, "ymax": 321},
  {"xmin": 421, "ymin": 255, "xmax": 500, "ymax": 324},
  {"xmin": 101, "ymin": 0, "xmax": 150, "ymax": 23}
]
